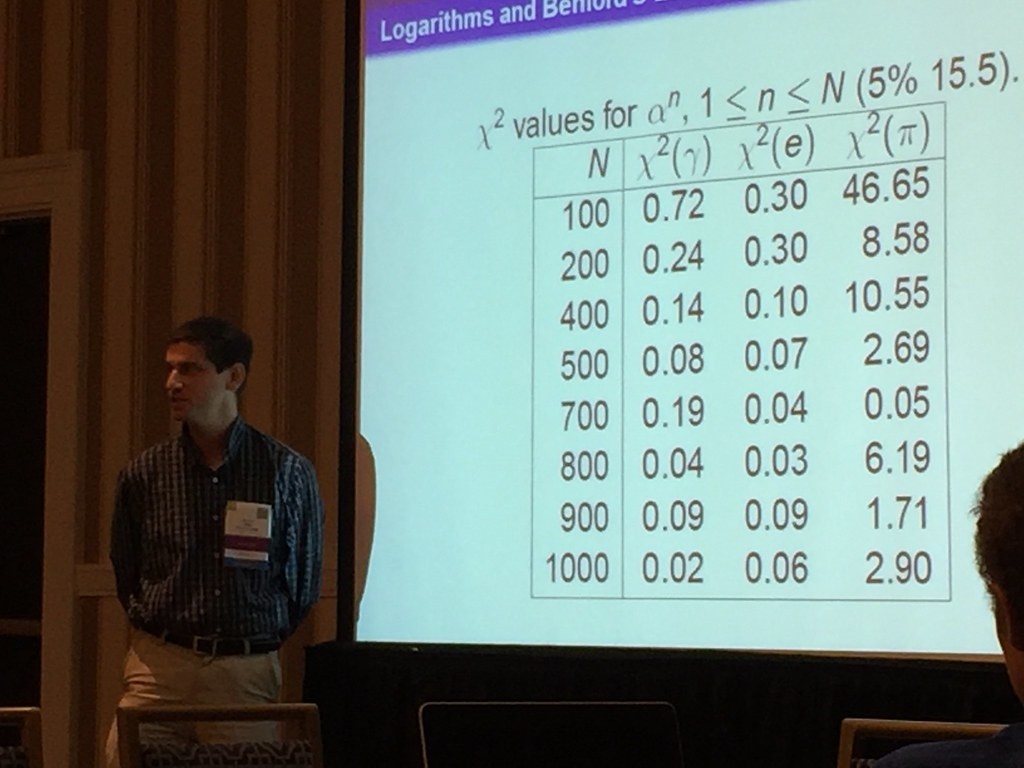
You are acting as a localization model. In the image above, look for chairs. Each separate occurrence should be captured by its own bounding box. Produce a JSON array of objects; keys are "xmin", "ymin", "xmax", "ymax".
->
[{"xmin": 0, "ymin": 702, "xmax": 1010, "ymax": 768}]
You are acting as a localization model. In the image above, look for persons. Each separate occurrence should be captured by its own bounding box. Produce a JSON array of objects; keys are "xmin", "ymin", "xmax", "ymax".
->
[
  {"xmin": 870, "ymin": 441, "xmax": 1024, "ymax": 768},
  {"xmin": 106, "ymin": 316, "xmax": 321, "ymax": 768}
]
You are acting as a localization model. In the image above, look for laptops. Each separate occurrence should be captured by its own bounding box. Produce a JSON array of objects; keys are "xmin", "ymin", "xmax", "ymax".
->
[{"xmin": 419, "ymin": 700, "xmax": 685, "ymax": 768}]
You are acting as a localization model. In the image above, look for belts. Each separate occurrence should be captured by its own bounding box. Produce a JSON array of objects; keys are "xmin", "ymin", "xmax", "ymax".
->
[{"xmin": 141, "ymin": 621, "xmax": 282, "ymax": 657}]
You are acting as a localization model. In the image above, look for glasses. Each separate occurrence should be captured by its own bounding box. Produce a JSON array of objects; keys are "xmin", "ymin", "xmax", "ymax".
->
[{"xmin": 164, "ymin": 361, "xmax": 216, "ymax": 376}]
